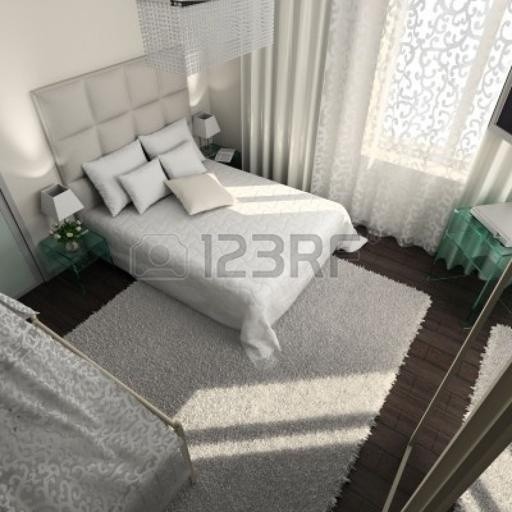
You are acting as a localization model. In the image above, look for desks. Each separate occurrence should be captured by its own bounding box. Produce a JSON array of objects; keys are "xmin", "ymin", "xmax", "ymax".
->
[
  {"xmin": 40, "ymin": 223, "xmax": 116, "ymax": 297},
  {"xmin": 427, "ymin": 205, "xmax": 511, "ymax": 330}
]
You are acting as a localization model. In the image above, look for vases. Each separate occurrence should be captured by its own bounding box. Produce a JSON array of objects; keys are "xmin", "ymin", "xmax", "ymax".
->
[{"xmin": 65, "ymin": 241, "xmax": 79, "ymax": 253}]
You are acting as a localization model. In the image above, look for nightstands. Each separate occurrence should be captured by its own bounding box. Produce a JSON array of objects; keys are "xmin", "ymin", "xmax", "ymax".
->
[{"xmin": 200, "ymin": 144, "xmax": 242, "ymax": 171}]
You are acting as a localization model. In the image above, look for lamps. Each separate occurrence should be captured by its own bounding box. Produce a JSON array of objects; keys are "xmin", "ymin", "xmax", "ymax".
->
[
  {"xmin": 191, "ymin": 110, "xmax": 221, "ymax": 159},
  {"xmin": 41, "ymin": 183, "xmax": 85, "ymax": 224}
]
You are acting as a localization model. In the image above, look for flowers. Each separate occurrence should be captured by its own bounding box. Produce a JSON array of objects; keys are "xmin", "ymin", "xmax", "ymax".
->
[{"xmin": 48, "ymin": 217, "xmax": 91, "ymax": 243}]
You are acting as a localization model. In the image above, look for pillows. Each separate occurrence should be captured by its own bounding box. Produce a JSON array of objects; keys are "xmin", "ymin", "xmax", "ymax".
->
[{"xmin": 82, "ymin": 117, "xmax": 238, "ymax": 219}]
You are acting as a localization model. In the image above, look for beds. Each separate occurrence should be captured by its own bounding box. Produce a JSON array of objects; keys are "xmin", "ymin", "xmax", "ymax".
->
[
  {"xmin": 1, "ymin": 292, "xmax": 197, "ymax": 511},
  {"xmin": 29, "ymin": 44, "xmax": 346, "ymax": 333}
]
